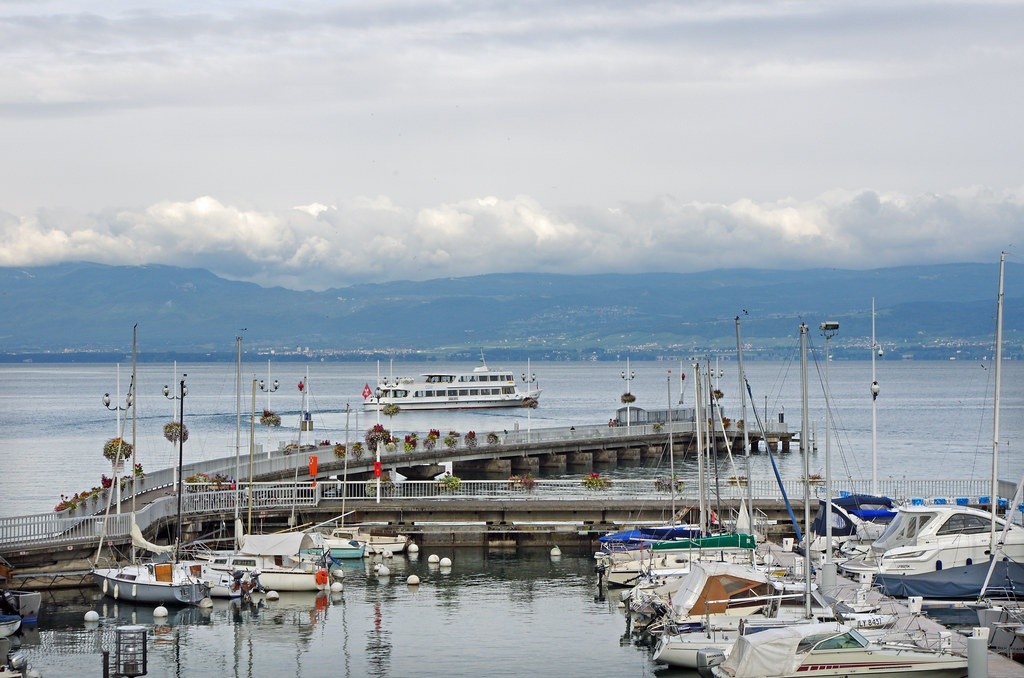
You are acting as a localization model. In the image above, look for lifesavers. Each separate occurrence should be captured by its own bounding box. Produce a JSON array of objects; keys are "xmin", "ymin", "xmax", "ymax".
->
[{"xmin": 316, "ymin": 571, "xmax": 327, "ymax": 585}]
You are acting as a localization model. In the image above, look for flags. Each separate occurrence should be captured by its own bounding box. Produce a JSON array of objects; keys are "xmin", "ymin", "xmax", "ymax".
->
[{"xmin": 362, "ymin": 385, "xmax": 371, "ymax": 399}]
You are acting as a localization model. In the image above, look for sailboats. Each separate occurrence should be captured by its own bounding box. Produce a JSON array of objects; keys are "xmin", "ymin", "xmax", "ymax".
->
[{"xmin": 0, "ymin": 308, "xmax": 1023, "ymax": 677}]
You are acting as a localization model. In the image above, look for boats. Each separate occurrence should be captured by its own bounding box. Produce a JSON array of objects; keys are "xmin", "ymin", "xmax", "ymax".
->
[{"xmin": 360, "ymin": 346, "xmax": 543, "ymax": 412}]
